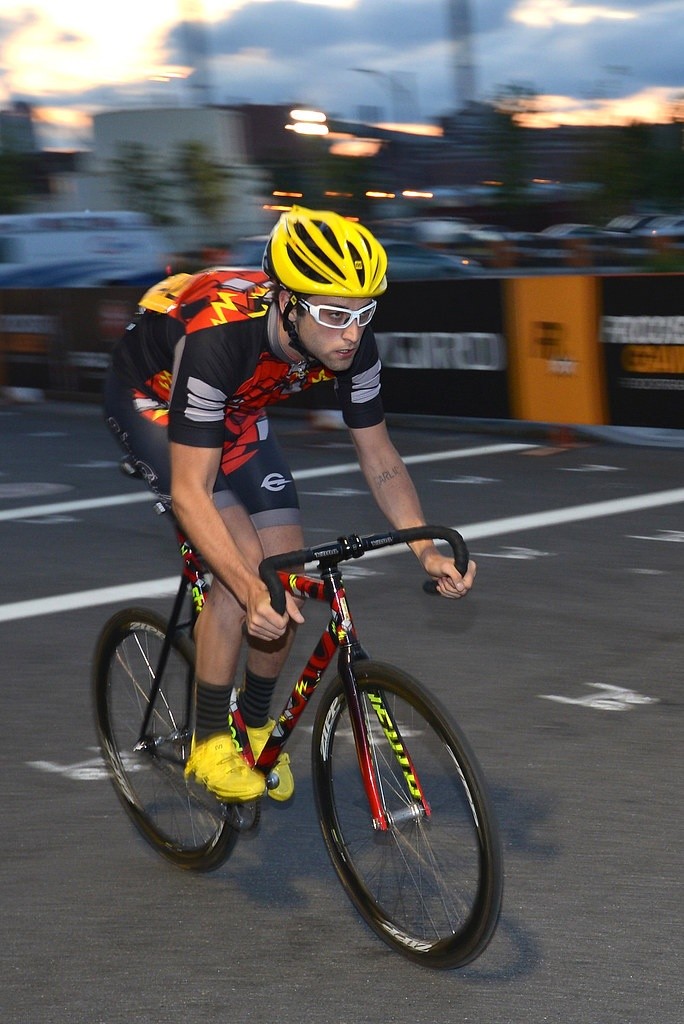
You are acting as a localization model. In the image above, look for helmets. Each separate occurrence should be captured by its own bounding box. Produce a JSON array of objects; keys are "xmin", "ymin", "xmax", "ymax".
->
[{"xmin": 260, "ymin": 204, "xmax": 389, "ymax": 297}]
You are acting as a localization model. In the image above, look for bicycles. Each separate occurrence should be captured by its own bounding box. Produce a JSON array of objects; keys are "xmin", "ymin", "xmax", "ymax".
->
[{"xmin": 87, "ymin": 452, "xmax": 505, "ymax": 972}]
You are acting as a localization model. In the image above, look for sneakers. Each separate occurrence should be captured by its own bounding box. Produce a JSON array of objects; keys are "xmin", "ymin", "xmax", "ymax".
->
[
  {"xmin": 235, "ymin": 687, "xmax": 295, "ymax": 801},
  {"xmin": 182, "ymin": 714, "xmax": 266, "ymax": 802}
]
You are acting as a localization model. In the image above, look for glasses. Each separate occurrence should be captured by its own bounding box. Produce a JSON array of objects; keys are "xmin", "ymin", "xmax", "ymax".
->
[{"xmin": 279, "ymin": 283, "xmax": 378, "ymax": 329}]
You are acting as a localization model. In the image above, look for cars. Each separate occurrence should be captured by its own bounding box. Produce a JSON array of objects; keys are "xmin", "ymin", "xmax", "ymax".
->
[{"xmin": 0, "ymin": 188, "xmax": 677, "ymax": 400}]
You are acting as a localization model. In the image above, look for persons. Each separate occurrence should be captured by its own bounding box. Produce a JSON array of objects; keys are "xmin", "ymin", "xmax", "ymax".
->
[{"xmin": 109, "ymin": 204, "xmax": 483, "ymax": 803}]
